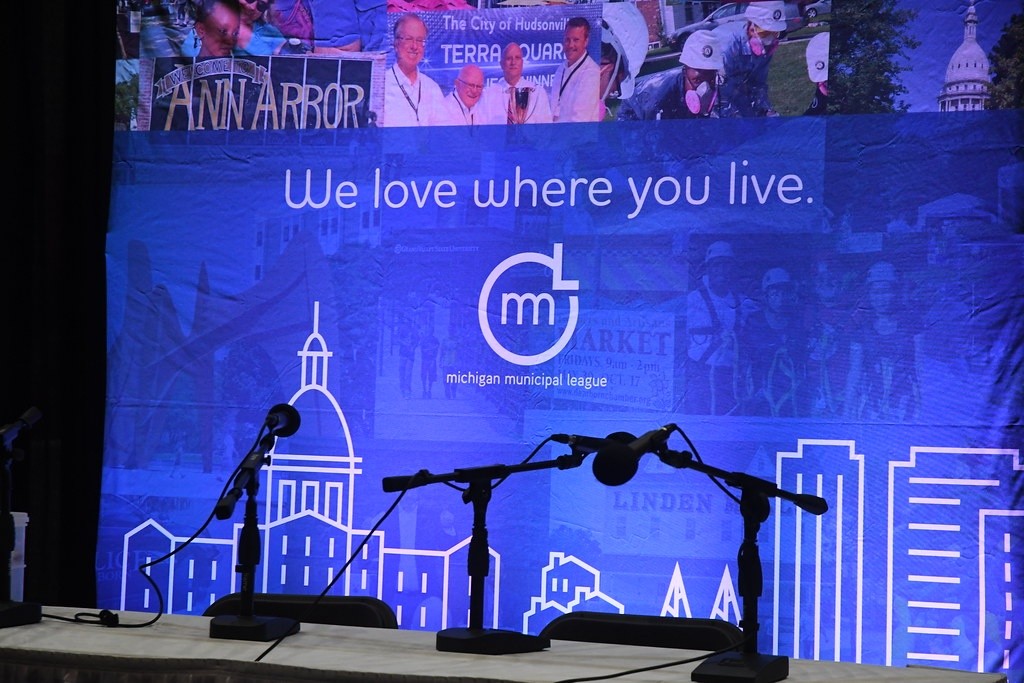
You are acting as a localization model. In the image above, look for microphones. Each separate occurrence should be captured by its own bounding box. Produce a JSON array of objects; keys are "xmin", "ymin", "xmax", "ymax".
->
[
  {"xmin": 593, "ymin": 423, "xmax": 677, "ymax": 486},
  {"xmin": 266, "ymin": 403, "xmax": 300, "ymax": 437},
  {"xmin": 549, "ymin": 432, "xmax": 638, "ymax": 452}
]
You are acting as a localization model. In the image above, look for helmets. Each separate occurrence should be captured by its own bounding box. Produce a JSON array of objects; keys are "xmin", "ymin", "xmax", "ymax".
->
[
  {"xmin": 602, "ymin": 3, "xmax": 649, "ymax": 81},
  {"xmin": 680, "ymin": 31, "xmax": 724, "ymax": 70}
]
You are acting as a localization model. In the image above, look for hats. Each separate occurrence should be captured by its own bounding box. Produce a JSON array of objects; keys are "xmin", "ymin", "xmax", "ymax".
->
[
  {"xmin": 746, "ymin": 2, "xmax": 787, "ymax": 30},
  {"xmin": 806, "ymin": 31, "xmax": 828, "ymax": 82}
]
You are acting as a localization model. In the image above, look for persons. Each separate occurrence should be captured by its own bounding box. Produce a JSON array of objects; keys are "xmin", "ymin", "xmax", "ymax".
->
[
  {"xmin": 182, "ymin": 0, "xmax": 287, "ymax": 57},
  {"xmin": 599, "ymin": 2, "xmax": 648, "ymax": 122},
  {"xmin": 475, "ymin": 40, "xmax": 552, "ymax": 123},
  {"xmin": 704, "ymin": 0, "xmax": 786, "ymax": 117},
  {"xmin": 444, "ymin": 63, "xmax": 484, "ymax": 125},
  {"xmin": 614, "ymin": 30, "xmax": 725, "ymax": 121},
  {"xmin": 381, "ymin": 11, "xmax": 444, "ymax": 126},
  {"xmin": 549, "ymin": 17, "xmax": 600, "ymax": 122},
  {"xmin": 798, "ymin": 32, "xmax": 830, "ymax": 115},
  {"xmin": 193, "ymin": 0, "xmax": 240, "ymax": 59}
]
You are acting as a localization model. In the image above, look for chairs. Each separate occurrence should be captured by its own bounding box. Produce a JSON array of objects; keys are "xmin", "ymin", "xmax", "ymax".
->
[
  {"xmin": 537, "ymin": 610, "xmax": 746, "ymax": 653},
  {"xmin": 201, "ymin": 592, "xmax": 398, "ymax": 629}
]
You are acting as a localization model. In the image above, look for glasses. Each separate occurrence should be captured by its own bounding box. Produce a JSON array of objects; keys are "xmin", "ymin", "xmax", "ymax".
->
[
  {"xmin": 457, "ymin": 77, "xmax": 483, "ymax": 91},
  {"xmin": 396, "ymin": 35, "xmax": 426, "ymax": 47},
  {"xmin": 205, "ymin": 23, "xmax": 241, "ymax": 39}
]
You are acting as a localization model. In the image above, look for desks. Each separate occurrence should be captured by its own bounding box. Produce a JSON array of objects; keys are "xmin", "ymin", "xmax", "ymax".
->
[{"xmin": 1, "ymin": 603, "xmax": 1013, "ymax": 682}]
